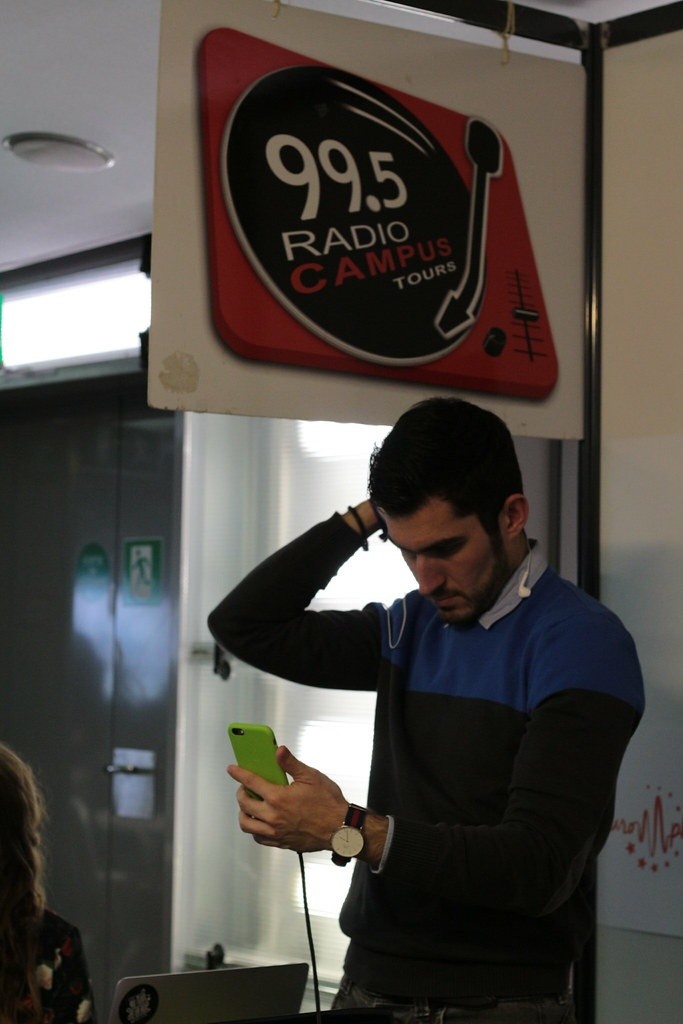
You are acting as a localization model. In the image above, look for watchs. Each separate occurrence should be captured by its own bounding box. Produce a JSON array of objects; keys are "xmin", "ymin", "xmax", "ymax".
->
[{"xmin": 327, "ymin": 803, "xmax": 369, "ymax": 870}]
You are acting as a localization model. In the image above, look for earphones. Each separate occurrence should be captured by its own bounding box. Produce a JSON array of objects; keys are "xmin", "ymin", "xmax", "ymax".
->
[{"xmin": 517, "ymin": 569, "xmax": 530, "ymax": 597}]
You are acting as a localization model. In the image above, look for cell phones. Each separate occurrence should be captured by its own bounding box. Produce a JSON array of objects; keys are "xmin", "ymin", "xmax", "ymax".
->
[{"xmin": 228, "ymin": 722, "xmax": 290, "ymax": 801}]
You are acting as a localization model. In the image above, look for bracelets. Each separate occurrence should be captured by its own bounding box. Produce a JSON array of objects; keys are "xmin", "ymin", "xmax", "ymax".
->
[{"xmin": 349, "ymin": 503, "xmax": 369, "ymax": 550}]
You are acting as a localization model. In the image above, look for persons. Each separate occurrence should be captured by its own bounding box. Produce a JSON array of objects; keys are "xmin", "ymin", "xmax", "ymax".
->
[
  {"xmin": 200, "ymin": 398, "xmax": 644, "ymax": 1024},
  {"xmin": 0, "ymin": 743, "xmax": 97, "ymax": 1022}
]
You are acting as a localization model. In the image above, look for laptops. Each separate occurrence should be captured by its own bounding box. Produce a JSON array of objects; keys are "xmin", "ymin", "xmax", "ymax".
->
[{"xmin": 107, "ymin": 962, "xmax": 309, "ymax": 1024}]
